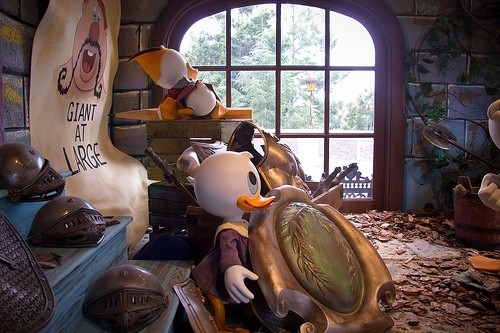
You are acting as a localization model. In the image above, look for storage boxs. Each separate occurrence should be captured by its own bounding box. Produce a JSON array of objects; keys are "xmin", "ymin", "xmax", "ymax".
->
[
  {"xmin": 145, "ymin": 117, "xmax": 254, "ymax": 183},
  {"xmin": 147, "ymin": 180, "xmax": 345, "ymax": 263}
]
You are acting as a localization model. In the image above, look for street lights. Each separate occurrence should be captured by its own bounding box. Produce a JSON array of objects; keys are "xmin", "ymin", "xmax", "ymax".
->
[{"xmin": 305, "ymin": 77, "xmax": 317, "ymax": 128}]
